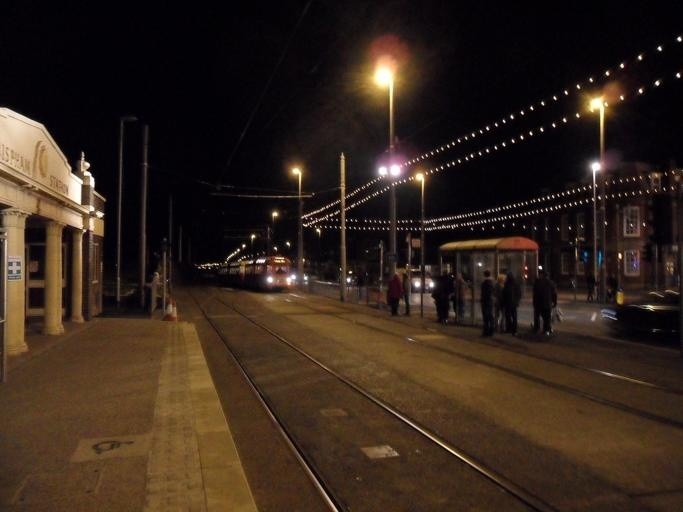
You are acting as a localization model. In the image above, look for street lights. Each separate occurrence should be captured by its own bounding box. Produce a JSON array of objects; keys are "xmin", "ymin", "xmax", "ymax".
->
[
  {"xmin": 292, "ymin": 168, "xmax": 304, "ymax": 293},
  {"xmin": 415, "ymin": 172, "xmax": 424, "ymax": 318},
  {"xmin": 591, "ymin": 162, "xmax": 601, "ymax": 303},
  {"xmin": 589, "ymin": 98, "xmax": 607, "ymax": 304},
  {"xmin": 374, "ymin": 65, "xmax": 398, "ymax": 269}
]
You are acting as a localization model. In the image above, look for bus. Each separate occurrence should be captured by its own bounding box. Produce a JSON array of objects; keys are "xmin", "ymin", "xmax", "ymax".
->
[{"xmin": 217, "ymin": 256, "xmax": 291, "ymax": 292}]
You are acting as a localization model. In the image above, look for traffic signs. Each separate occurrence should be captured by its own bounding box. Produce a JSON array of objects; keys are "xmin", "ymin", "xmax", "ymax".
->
[{"xmin": 385, "ymin": 254, "xmax": 398, "ymax": 263}]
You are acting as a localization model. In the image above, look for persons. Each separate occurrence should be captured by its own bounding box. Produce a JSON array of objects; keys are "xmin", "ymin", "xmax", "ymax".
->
[
  {"xmin": 388, "ymin": 272, "xmax": 403, "ymax": 316},
  {"xmin": 586, "ymin": 270, "xmax": 595, "ymax": 301},
  {"xmin": 430, "ymin": 267, "xmax": 557, "ymax": 339},
  {"xmin": 608, "ymin": 273, "xmax": 617, "ymax": 303},
  {"xmin": 402, "ymin": 273, "xmax": 410, "ymax": 315}
]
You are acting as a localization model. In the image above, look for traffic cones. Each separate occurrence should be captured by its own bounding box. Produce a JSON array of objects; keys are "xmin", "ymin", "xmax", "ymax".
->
[{"xmin": 163, "ymin": 297, "xmax": 177, "ymax": 322}]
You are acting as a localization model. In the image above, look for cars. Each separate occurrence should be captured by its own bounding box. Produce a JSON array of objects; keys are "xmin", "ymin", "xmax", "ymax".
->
[
  {"xmin": 401, "ymin": 269, "xmax": 435, "ymax": 294},
  {"xmin": 339, "ymin": 266, "xmax": 374, "ymax": 286}
]
